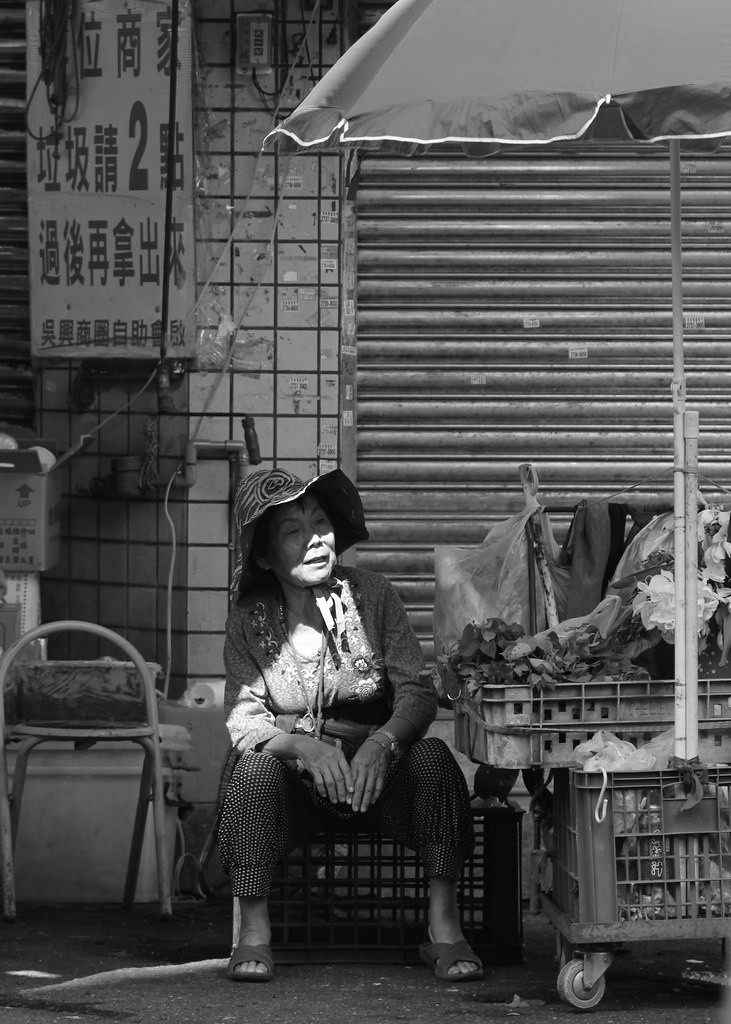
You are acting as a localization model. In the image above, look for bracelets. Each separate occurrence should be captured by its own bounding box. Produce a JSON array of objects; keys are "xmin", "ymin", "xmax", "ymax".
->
[{"xmin": 366, "ymin": 737, "xmax": 390, "ymax": 763}]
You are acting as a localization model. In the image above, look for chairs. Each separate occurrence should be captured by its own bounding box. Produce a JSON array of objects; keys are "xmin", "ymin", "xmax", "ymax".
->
[{"xmin": 0, "ymin": 624, "xmax": 173, "ymax": 923}]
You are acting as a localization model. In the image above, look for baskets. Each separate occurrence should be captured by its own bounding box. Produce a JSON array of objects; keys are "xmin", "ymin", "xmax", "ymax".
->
[{"xmin": 454, "ymin": 678, "xmax": 731, "ymax": 770}]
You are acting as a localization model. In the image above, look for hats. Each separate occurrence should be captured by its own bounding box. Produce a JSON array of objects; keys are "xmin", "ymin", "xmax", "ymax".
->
[{"xmin": 228, "ymin": 468, "xmax": 370, "ymax": 607}]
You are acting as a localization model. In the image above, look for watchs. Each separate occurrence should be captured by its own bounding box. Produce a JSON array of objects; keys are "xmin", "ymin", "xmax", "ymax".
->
[{"xmin": 373, "ymin": 730, "xmax": 399, "ymax": 755}]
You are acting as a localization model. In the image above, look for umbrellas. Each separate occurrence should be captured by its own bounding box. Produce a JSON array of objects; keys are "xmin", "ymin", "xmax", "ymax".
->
[{"xmin": 262, "ymin": 0, "xmax": 731, "ymax": 376}]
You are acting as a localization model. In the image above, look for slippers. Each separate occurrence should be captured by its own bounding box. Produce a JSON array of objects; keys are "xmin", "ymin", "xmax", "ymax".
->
[
  {"xmin": 227, "ymin": 944, "xmax": 275, "ymax": 980},
  {"xmin": 419, "ymin": 939, "xmax": 483, "ymax": 982}
]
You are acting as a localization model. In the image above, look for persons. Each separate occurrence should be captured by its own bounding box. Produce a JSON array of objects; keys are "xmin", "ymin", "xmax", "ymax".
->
[{"xmin": 215, "ymin": 468, "xmax": 483, "ymax": 982}]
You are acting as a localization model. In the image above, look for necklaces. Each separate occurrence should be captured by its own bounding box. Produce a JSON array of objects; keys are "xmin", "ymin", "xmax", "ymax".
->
[{"xmin": 277, "ymin": 598, "xmax": 329, "ymax": 741}]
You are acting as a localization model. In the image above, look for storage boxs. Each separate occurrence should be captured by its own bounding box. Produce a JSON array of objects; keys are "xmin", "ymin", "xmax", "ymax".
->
[
  {"xmin": 1, "ymin": 603, "xmax": 22, "ymax": 651},
  {"xmin": 270, "ymin": 799, "xmax": 523, "ymax": 966},
  {"xmin": 452, "ymin": 670, "xmax": 731, "ymax": 769},
  {"xmin": 31, "ymin": 637, "xmax": 48, "ymax": 661},
  {"xmin": 0, "ymin": 455, "xmax": 73, "ymax": 569},
  {"xmin": 552, "ymin": 769, "xmax": 731, "ymax": 926},
  {"xmin": 0, "ymin": 572, "xmax": 41, "ymax": 635}
]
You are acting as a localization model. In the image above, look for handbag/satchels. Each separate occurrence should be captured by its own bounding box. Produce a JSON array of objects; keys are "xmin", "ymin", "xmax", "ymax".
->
[{"xmin": 433, "ymin": 503, "xmax": 572, "ymax": 709}]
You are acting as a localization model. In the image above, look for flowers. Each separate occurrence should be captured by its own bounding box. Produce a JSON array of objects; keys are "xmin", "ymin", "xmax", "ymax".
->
[{"xmin": 636, "ymin": 517, "xmax": 731, "ymax": 647}]
